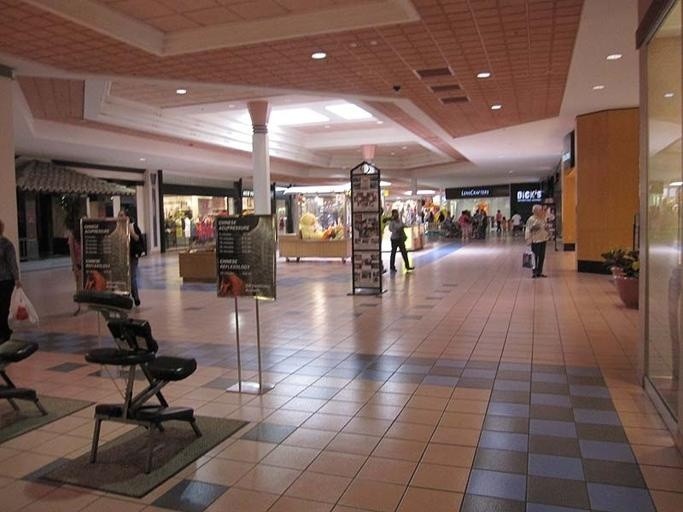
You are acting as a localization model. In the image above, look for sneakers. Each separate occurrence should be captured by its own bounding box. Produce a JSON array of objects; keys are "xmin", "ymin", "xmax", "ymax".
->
[
  {"xmin": 389, "ymin": 266, "xmax": 397, "ymax": 272},
  {"xmin": 406, "ymin": 266, "xmax": 415, "ymax": 270},
  {"xmin": 532, "ymin": 268, "xmax": 547, "ymax": 278}
]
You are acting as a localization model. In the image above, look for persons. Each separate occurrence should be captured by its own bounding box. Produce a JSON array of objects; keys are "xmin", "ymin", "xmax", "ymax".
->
[
  {"xmin": 116, "ymin": 206, "xmax": 146, "ymax": 307},
  {"xmin": 525, "ymin": 204, "xmax": 554, "ymax": 279},
  {"xmin": 389, "ymin": 208, "xmax": 415, "ymax": 272},
  {"xmin": 68, "ymin": 212, "xmax": 91, "ymax": 317},
  {"xmin": 423, "ymin": 210, "xmax": 433, "ymax": 223},
  {"xmin": 436, "ymin": 212, "xmax": 444, "ymax": 224},
  {"xmin": 0, "ymin": 220, "xmax": 20, "ymax": 348},
  {"xmin": 442, "ymin": 206, "xmax": 522, "ymax": 240},
  {"xmin": 547, "ymin": 208, "xmax": 555, "ymax": 241}
]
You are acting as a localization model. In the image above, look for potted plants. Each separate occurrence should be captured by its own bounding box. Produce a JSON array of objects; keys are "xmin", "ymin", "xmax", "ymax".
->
[{"xmin": 599, "ymin": 247, "xmax": 638, "ymax": 311}]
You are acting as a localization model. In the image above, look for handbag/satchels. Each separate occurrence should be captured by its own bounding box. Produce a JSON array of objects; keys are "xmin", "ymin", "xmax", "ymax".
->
[{"xmin": 523, "ymin": 253, "xmax": 532, "ymax": 267}]
